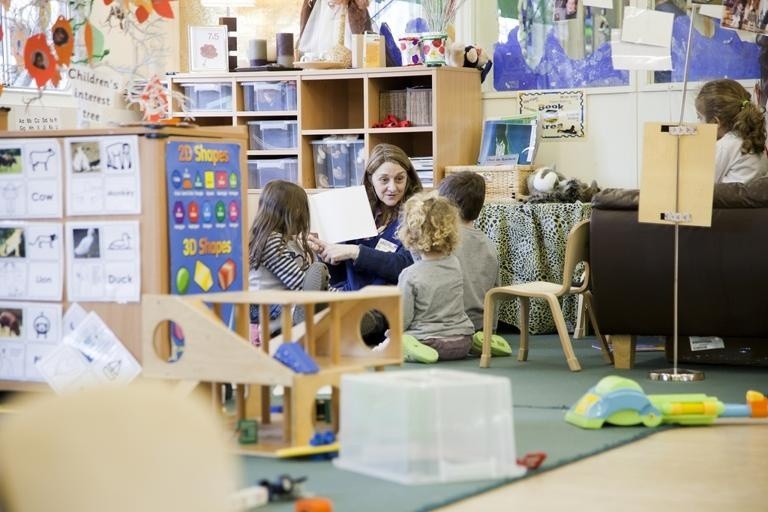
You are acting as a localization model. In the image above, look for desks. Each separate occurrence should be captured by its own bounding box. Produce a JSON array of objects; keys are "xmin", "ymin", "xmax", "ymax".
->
[{"xmin": 0, "ymin": 125, "xmax": 249, "ymax": 398}]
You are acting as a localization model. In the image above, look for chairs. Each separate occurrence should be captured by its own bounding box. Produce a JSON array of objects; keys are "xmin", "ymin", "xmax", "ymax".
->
[{"xmin": 478, "ymin": 217, "xmax": 613, "ymax": 372}]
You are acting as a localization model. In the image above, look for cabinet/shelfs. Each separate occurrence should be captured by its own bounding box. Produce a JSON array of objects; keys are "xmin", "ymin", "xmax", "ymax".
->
[{"xmin": 173, "ymin": 66, "xmax": 481, "ymax": 205}]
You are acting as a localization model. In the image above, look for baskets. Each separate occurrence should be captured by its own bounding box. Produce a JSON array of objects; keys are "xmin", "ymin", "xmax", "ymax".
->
[{"xmin": 443, "ymin": 164, "xmax": 537, "ymax": 204}]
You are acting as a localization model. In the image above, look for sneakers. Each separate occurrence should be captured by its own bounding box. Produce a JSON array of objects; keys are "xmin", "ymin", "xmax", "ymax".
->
[
  {"xmin": 471, "ymin": 330, "xmax": 514, "ymax": 357},
  {"xmin": 398, "ymin": 334, "xmax": 440, "ymax": 364}
]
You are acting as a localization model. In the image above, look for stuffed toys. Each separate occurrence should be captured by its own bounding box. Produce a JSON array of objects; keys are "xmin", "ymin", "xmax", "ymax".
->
[
  {"xmin": 450, "ymin": 43, "xmax": 489, "ymax": 68},
  {"xmin": 526, "ymin": 167, "xmax": 598, "ymax": 201}
]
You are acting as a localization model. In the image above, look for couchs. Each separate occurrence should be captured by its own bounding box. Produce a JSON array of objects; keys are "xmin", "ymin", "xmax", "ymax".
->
[{"xmin": 589, "ymin": 178, "xmax": 767, "ymax": 340}]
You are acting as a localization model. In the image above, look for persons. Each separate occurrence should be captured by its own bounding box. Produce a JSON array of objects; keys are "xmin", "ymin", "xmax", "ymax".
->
[
  {"xmin": 292, "ymin": 142, "xmax": 423, "ymax": 345},
  {"xmin": 695, "ymin": 79, "xmax": 768, "ymax": 184},
  {"xmin": 247, "ymin": 179, "xmax": 345, "ymax": 322},
  {"xmin": 372, "ymin": 192, "xmax": 512, "ymax": 363},
  {"xmin": 437, "ymin": 170, "xmax": 500, "ymax": 332}
]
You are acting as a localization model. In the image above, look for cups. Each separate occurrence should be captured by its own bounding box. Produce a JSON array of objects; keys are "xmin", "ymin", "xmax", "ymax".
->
[{"xmin": 247, "ymin": 38, "xmax": 267, "ymax": 66}]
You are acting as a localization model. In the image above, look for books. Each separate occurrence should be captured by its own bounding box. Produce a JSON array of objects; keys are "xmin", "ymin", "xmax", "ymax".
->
[{"xmin": 409, "ymin": 157, "xmax": 433, "ymax": 188}]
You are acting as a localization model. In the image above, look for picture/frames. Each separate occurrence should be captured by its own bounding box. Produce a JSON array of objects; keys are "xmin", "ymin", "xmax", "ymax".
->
[{"xmin": 478, "ymin": 118, "xmax": 541, "ymax": 168}]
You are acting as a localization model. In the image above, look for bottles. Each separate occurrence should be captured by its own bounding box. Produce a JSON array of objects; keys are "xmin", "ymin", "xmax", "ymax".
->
[{"xmin": 275, "ymin": 33, "xmax": 293, "ymax": 70}]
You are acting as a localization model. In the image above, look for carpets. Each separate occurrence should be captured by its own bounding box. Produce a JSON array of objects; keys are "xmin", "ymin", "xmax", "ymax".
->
[{"xmin": 232, "ymin": 327, "xmax": 766, "ymax": 512}]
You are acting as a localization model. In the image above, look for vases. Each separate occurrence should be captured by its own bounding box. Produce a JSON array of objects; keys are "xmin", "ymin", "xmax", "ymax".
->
[
  {"xmin": 395, "ymin": 35, "xmax": 422, "ymax": 67},
  {"xmin": 421, "ymin": 32, "xmax": 450, "ymax": 68}
]
